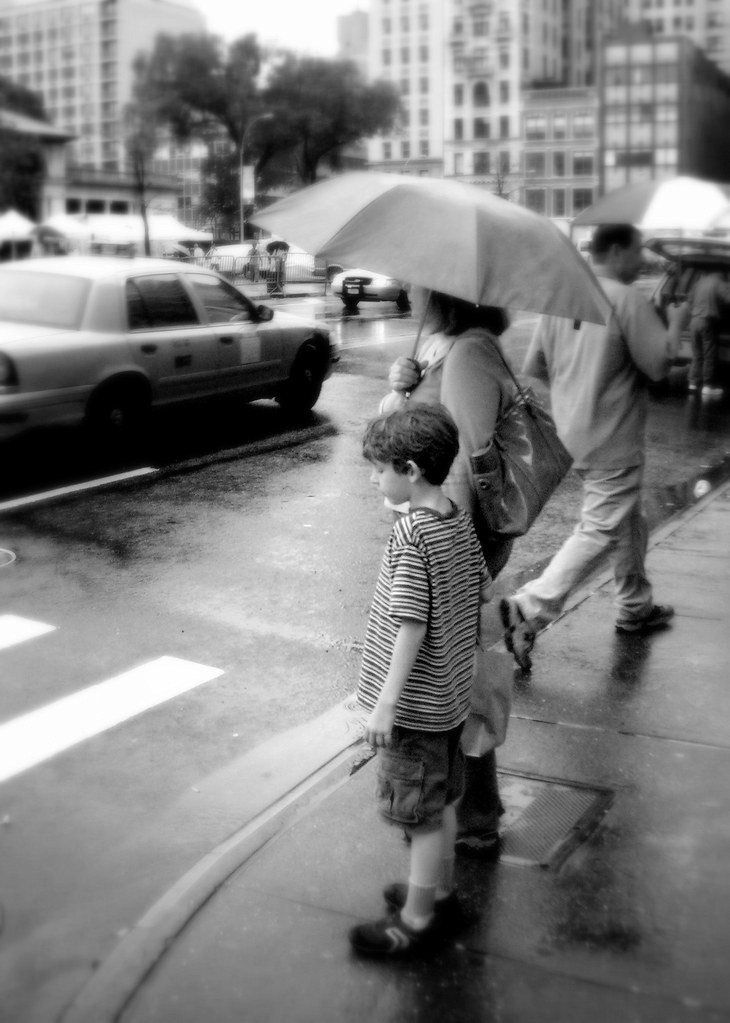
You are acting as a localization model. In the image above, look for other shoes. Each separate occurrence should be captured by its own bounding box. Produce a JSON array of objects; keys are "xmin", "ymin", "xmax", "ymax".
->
[
  {"xmin": 403, "ymin": 825, "xmax": 500, "ymax": 854},
  {"xmin": 688, "ymin": 384, "xmax": 698, "ymax": 391},
  {"xmin": 701, "ymin": 386, "xmax": 723, "ymax": 395}
]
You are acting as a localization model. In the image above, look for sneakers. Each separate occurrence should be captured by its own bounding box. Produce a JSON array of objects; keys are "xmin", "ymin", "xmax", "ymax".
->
[
  {"xmin": 497, "ymin": 597, "xmax": 536, "ymax": 671},
  {"xmin": 614, "ymin": 602, "xmax": 675, "ymax": 633},
  {"xmin": 348, "ymin": 911, "xmax": 455, "ymax": 963},
  {"xmin": 382, "ymin": 881, "xmax": 480, "ymax": 930}
]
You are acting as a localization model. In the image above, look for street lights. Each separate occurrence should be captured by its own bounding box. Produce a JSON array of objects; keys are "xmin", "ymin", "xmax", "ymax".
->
[{"xmin": 240, "ymin": 113, "xmax": 277, "ymax": 241}]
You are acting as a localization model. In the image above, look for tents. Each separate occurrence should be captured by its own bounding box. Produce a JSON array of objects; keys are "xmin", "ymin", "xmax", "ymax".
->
[{"xmin": 0, "ymin": 207, "xmax": 215, "ymax": 259}]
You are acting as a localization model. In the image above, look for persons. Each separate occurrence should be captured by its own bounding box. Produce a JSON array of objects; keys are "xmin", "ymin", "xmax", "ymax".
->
[
  {"xmin": 206, "ymin": 243, "xmax": 221, "ymax": 274},
  {"xmin": 272, "ymin": 244, "xmax": 287, "ymax": 287},
  {"xmin": 193, "ymin": 243, "xmax": 206, "ymax": 267},
  {"xmin": 348, "ymin": 402, "xmax": 490, "ymax": 958},
  {"xmin": 247, "ymin": 240, "xmax": 263, "ymax": 281},
  {"xmin": 497, "ymin": 224, "xmax": 694, "ymax": 669},
  {"xmin": 377, "ymin": 281, "xmax": 515, "ymax": 867},
  {"xmin": 685, "ymin": 269, "xmax": 730, "ymax": 395}
]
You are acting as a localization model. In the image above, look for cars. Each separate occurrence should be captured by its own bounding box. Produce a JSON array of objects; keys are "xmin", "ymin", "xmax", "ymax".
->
[
  {"xmin": 210, "ymin": 234, "xmax": 323, "ymax": 283},
  {"xmin": 329, "ymin": 266, "xmax": 414, "ymax": 312},
  {"xmin": 0, "ymin": 258, "xmax": 348, "ymax": 472},
  {"xmin": 643, "ymin": 236, "xmax": 730, "ymax": 398}
]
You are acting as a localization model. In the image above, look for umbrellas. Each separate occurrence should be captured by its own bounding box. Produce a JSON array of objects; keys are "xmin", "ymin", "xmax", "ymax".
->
[
  {"xmin": 242, "ymin": 170, "xmax": 615, "ymax": 359},
  {"xmin": 266, "ymin": 241, "xmax": 291, "ymax": 253},
  {"xmin": 572, "ymin": 171, "xmax": 730, "ymax": 234}
]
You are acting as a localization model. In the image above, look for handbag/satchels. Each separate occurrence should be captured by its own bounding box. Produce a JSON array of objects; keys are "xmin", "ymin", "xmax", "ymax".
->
[
  {"xmin": 447, "ymin": 331, "xmax": 575, "ymax": 538},
  {"xmin": 458, "ymin": 634, "xmax": 512, "ymax": 758}
]
